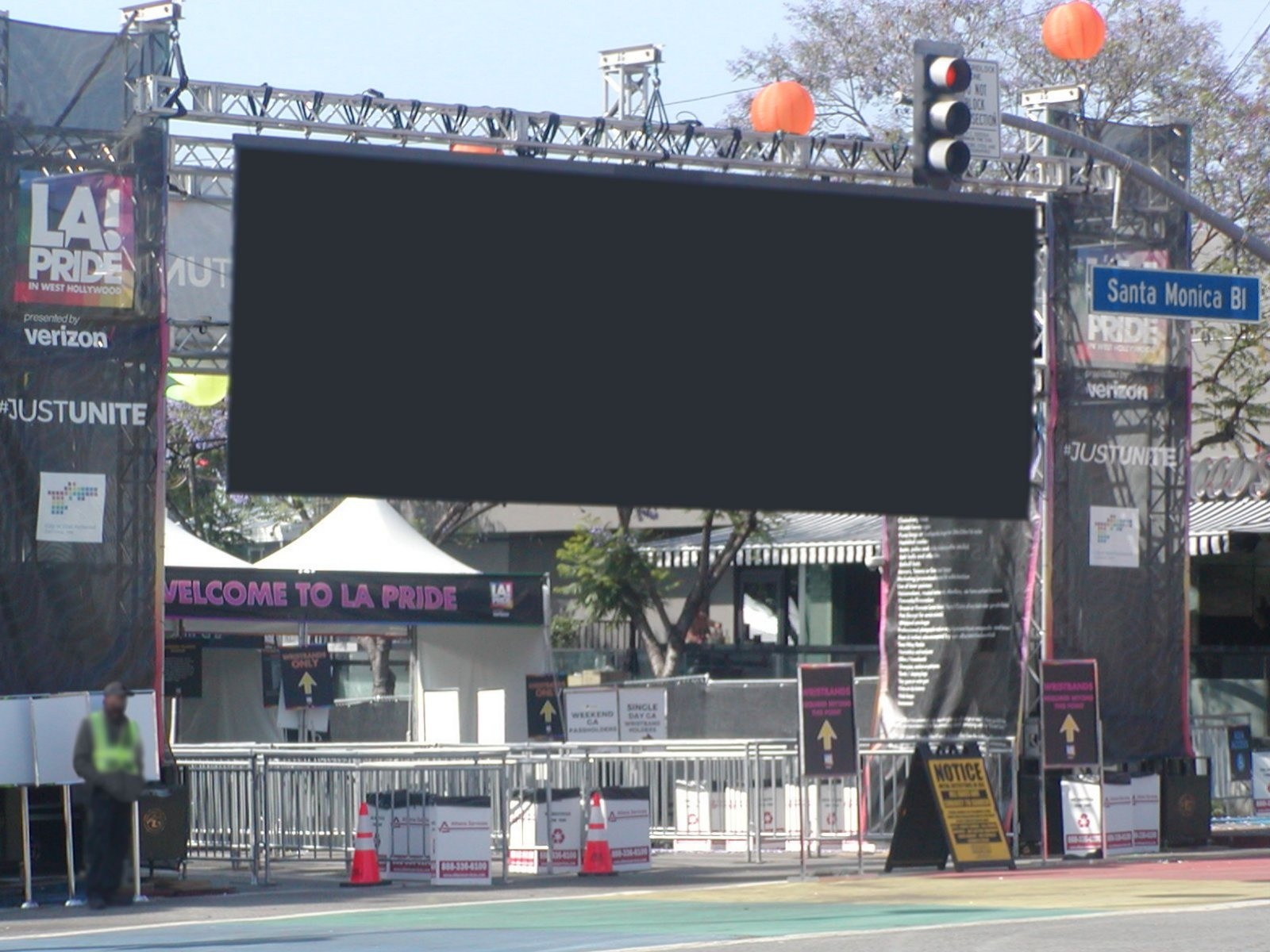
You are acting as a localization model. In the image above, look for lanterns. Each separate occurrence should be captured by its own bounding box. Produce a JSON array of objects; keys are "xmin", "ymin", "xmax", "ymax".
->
[
  {"xmin": 1042, "ymin": 2, "xmax": 1105, "ymax": 60},
  {"xmin": 751, "ymin": 78, "xmax": 813, "ymax": 135}
]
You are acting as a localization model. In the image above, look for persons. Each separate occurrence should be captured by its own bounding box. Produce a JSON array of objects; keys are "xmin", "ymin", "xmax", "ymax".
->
[
  {"xmin": 73, "ymin": 681, "xmax": 143, "ymax": 908},
  {"xmin": 687, "ymin": 609, "xmax": 724, "ymax": 643}
]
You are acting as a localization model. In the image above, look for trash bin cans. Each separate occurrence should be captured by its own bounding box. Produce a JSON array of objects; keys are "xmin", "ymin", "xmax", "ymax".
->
[
  {"xmin": 1148, "ymin": 755, "xmax": 1213, "ymax": 849},
  {"xmin": 138, "ymin": 783, "xmax": 190, "ymax": 868}
]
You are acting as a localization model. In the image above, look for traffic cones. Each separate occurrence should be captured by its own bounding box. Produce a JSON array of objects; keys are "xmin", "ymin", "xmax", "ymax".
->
[
  {"xmin": 339, "ymin": 802, "xmax": 393, "ymax": 887},
  {"xmin": 577, "ymin": 793, "xmax": 620, "ymax": 876}
]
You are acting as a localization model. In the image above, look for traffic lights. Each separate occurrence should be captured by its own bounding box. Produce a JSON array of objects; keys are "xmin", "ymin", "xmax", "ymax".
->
[{"xmin": 910, "ymin": 39, "xmax": 974, "ymax": 186}]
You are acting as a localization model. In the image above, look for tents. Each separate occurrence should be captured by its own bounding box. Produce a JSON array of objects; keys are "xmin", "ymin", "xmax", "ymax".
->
[{"xmin": 165, "ymin": 495, "xmax": 569, "ymax": 741}]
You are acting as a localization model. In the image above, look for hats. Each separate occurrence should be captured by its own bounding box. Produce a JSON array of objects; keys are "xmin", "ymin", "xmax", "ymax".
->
[{"xmin": 104, "ymin": 681, "xmax": 130, "ymax": 696}]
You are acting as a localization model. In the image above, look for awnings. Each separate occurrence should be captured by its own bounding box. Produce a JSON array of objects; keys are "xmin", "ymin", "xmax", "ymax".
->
[{"xmin": 638, "ymin": 499, "xmax": 1270, "ymax": 567}]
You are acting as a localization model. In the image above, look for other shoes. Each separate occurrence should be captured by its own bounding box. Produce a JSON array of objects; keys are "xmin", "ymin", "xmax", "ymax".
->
[
  {"xmin": 105, "ymin": 895, "xmax": 131, "ymax": 906},
  {"xmin": 88, "ymin": 896, "xmax": 106, "ymax": 908}
]
